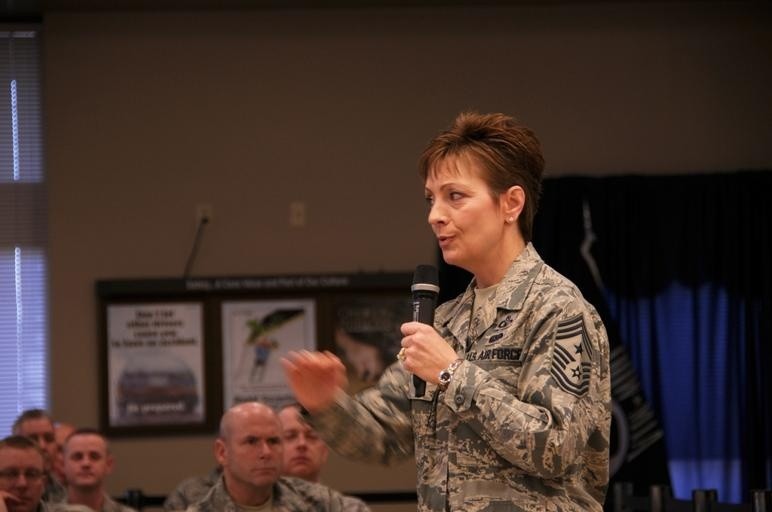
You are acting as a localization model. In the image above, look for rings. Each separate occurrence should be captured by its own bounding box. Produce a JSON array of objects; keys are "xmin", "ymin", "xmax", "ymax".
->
[{"xmin": 397, "ymin": 346, "xmax": 405, "ymax": 362}]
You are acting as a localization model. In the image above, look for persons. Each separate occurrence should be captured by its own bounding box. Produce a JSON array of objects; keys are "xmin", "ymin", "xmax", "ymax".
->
[
  {"xmin": 0, "ymin": 408, "xmax": 140, "ymax": 512},
  {"xmin": 159, "ymin": 403, "xmax": 373, "ymax": 512},
  {"xmin": 279, "ymin": 402, "xmax": 331, "ymax": 487},
  {"xmin": 280, "ymin": 109, "xmax": 613, "ymax": 512}
]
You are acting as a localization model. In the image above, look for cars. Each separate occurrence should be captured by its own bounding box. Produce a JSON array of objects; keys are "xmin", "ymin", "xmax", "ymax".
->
[{"xmin": 116, "ymin": 352, "xmax": 199, "ymax": 416}]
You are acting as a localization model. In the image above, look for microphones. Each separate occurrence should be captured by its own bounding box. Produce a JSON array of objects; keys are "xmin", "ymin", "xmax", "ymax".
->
[{"xmin": 410, "ymin": 264, "xmax": 441, "ymax": 397}]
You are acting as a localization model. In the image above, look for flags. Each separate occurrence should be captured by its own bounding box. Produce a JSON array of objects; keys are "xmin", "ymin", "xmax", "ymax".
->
[{"xmin": 583, "ymin": 231, "xmax": 663, "ymax": 475}]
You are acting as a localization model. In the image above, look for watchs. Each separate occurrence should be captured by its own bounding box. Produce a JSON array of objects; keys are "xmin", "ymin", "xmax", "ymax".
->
[{"xmin": 437, "ymin": 355, "xmax": 465, "ymax": 394}]
